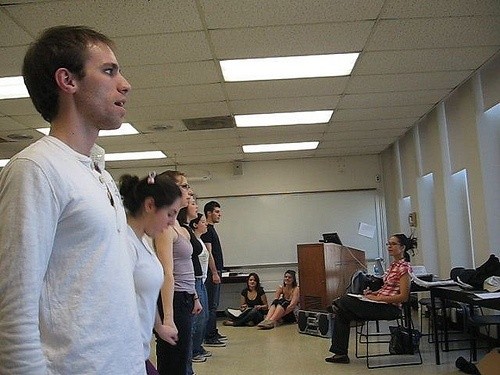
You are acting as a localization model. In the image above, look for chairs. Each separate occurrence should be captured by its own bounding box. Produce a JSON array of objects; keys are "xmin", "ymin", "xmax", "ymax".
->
[
  {"xmin": 419, "ymin": 298, "xmax": 442, "ymax": 335},
  {"xmin": 426, "ymin": 301, "xmax": 461, "ymax": 343},
  {"xmin": 355, "ymin": 282, "xmax": 423, "ymax": 369},
  {"xmin": 470, "ymin": 313, "xmax": 500, "ymax": 362}
]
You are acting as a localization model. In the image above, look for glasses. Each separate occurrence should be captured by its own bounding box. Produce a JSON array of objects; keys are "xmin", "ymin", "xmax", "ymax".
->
[
  {"xmin": 180, "ymin": 184, "xmax": 191, "ymax": 188},
  {"xmin": 386, "ymin": 242, "xmax": 402, "ymax": 246}
]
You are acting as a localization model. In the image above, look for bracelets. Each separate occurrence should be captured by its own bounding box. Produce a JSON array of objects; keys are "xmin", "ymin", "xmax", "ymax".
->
[{"xmin": 194, "ymin": 298, "xmax": 198, "ymax": 300}]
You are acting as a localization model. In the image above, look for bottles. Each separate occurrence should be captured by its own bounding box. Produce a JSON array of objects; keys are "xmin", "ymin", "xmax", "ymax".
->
[{"xmin": 373, "ymin": 258, "xmax": 381, "ymax": 278}]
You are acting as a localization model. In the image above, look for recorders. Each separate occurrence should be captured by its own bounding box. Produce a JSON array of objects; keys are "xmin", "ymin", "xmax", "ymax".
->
[{"xmin": 298, "ymin": 310, "xmax": 336, "ymax": 337}]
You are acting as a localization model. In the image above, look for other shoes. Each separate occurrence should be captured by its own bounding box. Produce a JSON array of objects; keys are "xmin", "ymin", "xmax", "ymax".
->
[
  {"xmin": 209, "ymin": 329, "xmax": 227, "ymax": 340},
  {"xmin": 258, "ymin": 319, "xmax": 268, "ymax": 327},
  {"xmin": 325, "ymin": 355, "xmax": 350, "ymax": 363},
  {"xmin": 202, "ymin": 333, "xmax": 226, "ymax": 347},
  {"xmin": 222, "ymin": 320, "xmax": 232, "ymax": 326},
  {"xmin": 261, "ymin": 321, "xmax": 274, "ymax": 328},
  {"xmin": 327, "ymin": 305, "xmax": 337, "ymax": 313},
  {"xmin": 192, "ymin": 356, "xmax": 206, "ymax": 362},
  {"xmin": 248, "ymin": 321, "xmax": 254, "ymax": 326},
  {"xmin": 199, "ymin": 347, "xmax": 211, "ymax": 356},
  {"xmin": 193, "ymin": 373, "xmax": 196, "ymax": 374}
]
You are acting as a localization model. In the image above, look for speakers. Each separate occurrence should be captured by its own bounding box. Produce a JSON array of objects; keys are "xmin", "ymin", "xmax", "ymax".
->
[{"xmin": 233, "ymin": 161, "xmax": 246, "ymax": 175}]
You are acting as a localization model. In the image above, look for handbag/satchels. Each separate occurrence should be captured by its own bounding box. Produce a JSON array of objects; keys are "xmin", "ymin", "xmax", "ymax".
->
[
  {"xmin": 346, "ymin": 271, "xmax": 384, "ymax": 294},
  {"xmin": 390, "ymin": 316, "xmax": 419, "ymax": 355}
]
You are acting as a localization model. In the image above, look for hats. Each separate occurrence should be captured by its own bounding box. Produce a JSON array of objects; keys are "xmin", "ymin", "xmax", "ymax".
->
[{"xmin": 484, "ymin": 276, "xmax": 500, "ymax": 292}]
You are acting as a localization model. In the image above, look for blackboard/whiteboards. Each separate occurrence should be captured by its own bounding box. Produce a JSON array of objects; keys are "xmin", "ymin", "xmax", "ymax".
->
[{"xmin": 197, "ymin": 187, "xmax": 381, "ymax": 270}]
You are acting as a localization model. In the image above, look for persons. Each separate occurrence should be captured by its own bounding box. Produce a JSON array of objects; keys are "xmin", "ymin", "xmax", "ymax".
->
[
  {"xmin": 189, "ymin": 212, "xmax": 212, "ymax": 362},
  {"xmin": 176, "ymin": 194, "xmax": 203, "ymax": 375},
  {"xmin": 153, "ymin": 169, "xmax": 203, "ymax": 375},
  {"xmin": 201, "ymin": 201, "xmax": 228, "ymax": 346},
  {"xmin": 0, "ymin": 25, "xmax": 147, "ymax": 375},
  {"xmin": 257, "ymin": 270, "xmax": 300, "ymax": 328},
  {"xmin": 119, "ymin": 171, "xmax": 183, "ymax": 375},
  {"xmin": 325, "ymin": 234, "xmax": 417, "ymax": 364},
  {"xmin": 223, "ymin": 272, "xmax": 268, "ymax": 326}
]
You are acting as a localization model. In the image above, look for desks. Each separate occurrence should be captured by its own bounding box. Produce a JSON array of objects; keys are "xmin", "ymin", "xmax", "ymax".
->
[{"xmin": 430, "ymin": 283, "xmax": 500, "ymax": 367}]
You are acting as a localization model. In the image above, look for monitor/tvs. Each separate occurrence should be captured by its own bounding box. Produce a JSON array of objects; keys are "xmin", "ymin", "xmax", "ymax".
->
[{"xmin": 323, "ymin": 233, "xmax": 342, "ymax": 246}]
